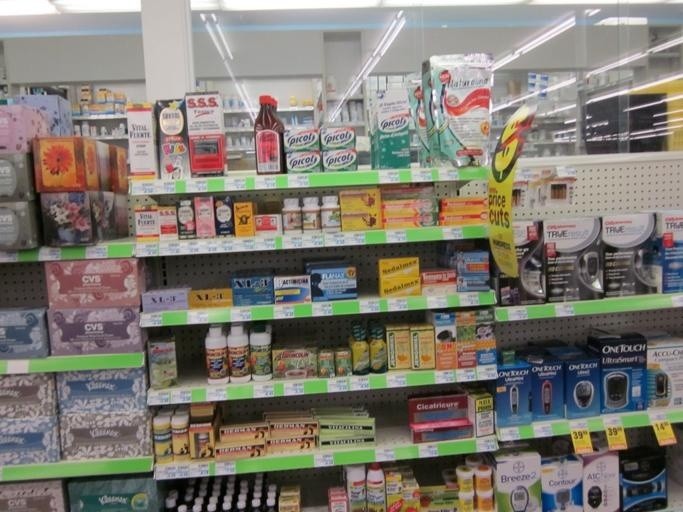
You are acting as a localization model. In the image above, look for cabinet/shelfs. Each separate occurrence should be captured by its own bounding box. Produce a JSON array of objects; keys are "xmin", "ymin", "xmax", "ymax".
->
[
  {"xmin": 137, "ymin": 152, "xmax": 497, "ymax": 511},
  {"xmin": 195, "ymin": 73, "xmax": 325, "ymax": 151},
  {"xmin": 496, "ymin": 153, "xmax": 683, "ymax": 512},
  {"xmin": 490, "ymin": 70, "xmax": 633, "ymax": 156},
  {"xmin": 0, "ymin": 240, "xmax": 153, "ymax": 512},
  {"xmin": 326, "ymin": 94, "xmax": 366, "ymax": 152},
  {"xmin": 0, "ymin": 77, "xmax": 144, "ymax": 163}
]
